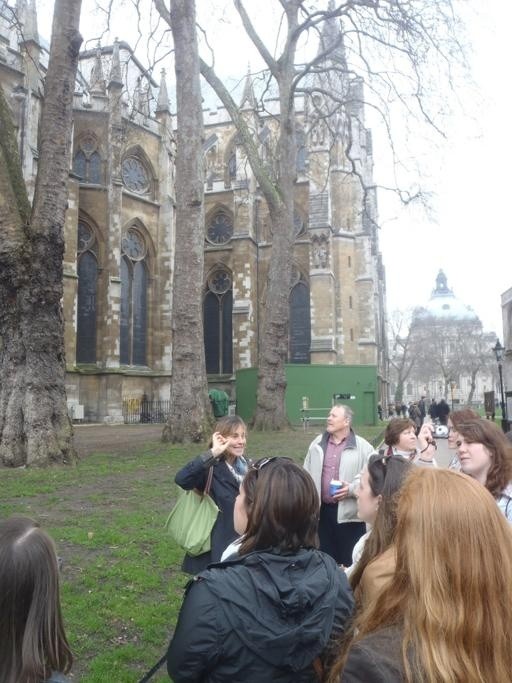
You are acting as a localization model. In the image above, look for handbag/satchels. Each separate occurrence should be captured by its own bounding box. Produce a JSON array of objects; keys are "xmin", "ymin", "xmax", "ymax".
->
[{"xmin": 164, "ymin": 466, "xmax": 220, "ymax": 558}]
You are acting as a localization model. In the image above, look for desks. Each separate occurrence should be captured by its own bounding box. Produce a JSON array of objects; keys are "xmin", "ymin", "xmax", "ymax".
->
[{"xmin": 299, "ymin": 407, "xmax": 331, "ymax": 433}]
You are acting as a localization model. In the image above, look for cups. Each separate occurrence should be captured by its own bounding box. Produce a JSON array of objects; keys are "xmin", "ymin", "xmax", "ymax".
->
[{"xmin": 330, "ymin": 480, "xmax": 343, "ymax": 497}]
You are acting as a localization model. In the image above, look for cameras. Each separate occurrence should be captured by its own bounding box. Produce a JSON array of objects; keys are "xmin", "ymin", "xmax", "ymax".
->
[{"xmin": 432, "ymin": 424, "xmax": 450, "ymax": 440}]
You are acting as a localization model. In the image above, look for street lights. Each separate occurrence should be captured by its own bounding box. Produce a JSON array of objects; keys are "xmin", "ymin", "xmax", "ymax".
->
[{"xmin": 490, "ymin": 339, "xmax": 509, "ymax": 432}]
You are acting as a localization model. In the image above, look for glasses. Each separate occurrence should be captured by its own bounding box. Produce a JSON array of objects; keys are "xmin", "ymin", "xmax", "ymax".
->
[{"xmin": 252, "ymin": 456, "xmax": 291, "ymax": 480}]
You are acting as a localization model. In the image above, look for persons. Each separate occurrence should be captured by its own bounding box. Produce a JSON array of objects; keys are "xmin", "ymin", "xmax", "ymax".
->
[
  {"xmin": 0, "ymin": 517, "xmax": 72, "ymax": 683},
  {"xmin": 168, "ymin": 456, "xmax": 355, "ymax": 683},
  {"xmin": 302, "ymin": 402, "xmax": 375, "ymax": 569},
  {"xmin": 417, "ymin": 408, "xmax": 512, "ymax": 523},
  {"xmin": 176, "ymin": 414, "xmax": 255, "ymax": 577},
  {"xmin": 329, "ymin": 467, "xmax": 512, "ymax": 683},
  {"xmin": 380, "ymin": 416, "xmax": 439, "ymax": 468},
  {"xmin": 377, "ymin": 397, "xmax": 450, "ymax": 437},
  {"xmin": 338, "ymin": 454, "xmax": 417, "ymax": 618}
]
